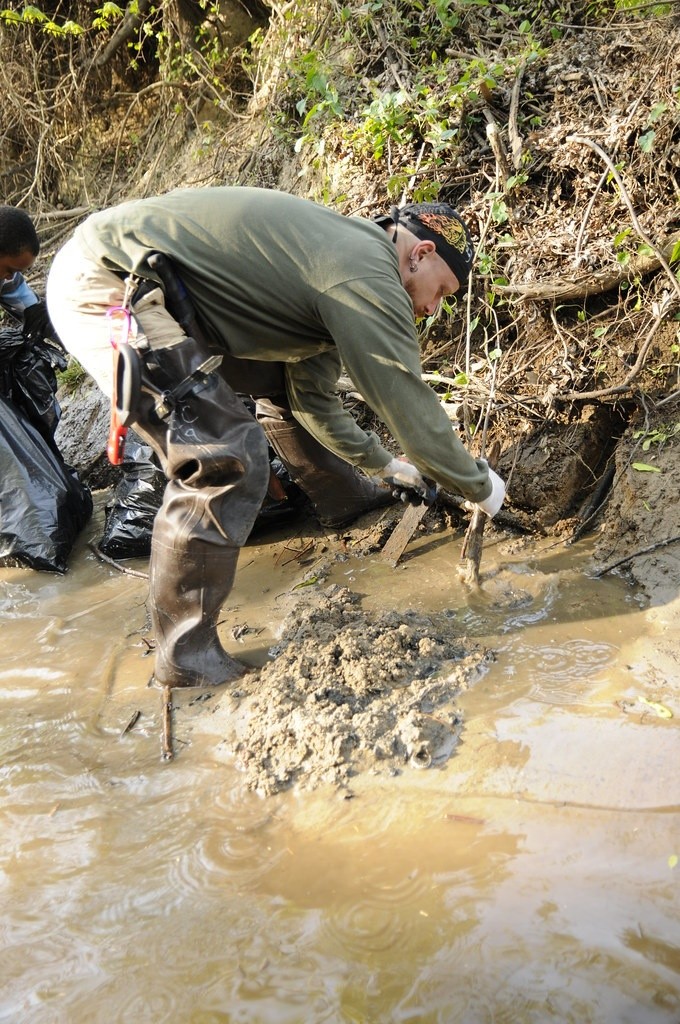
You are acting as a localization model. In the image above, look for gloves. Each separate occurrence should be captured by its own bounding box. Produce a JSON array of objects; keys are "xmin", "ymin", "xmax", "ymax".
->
[
  {"xmin": 464, "ymin": 467, "xmax": 505, "ymax": 518},
  {"xmin": 377, "ymin": 460, "xmax": 437, "ymax": 506}
]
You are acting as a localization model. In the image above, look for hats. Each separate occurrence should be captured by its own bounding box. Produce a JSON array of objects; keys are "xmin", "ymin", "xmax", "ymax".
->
[{"xmin": 376, "ymin": 202, "xmax": 475, "ymax": 292}]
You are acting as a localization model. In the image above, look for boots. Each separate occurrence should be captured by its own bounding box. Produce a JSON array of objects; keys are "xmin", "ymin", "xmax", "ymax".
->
[
  {"xmin": 218, "ymin": 358, "xmax": 397, "ymax": 529},
  {"xmin": 101, "ymin": 336, "xmax": 270, "ymax": 689}
]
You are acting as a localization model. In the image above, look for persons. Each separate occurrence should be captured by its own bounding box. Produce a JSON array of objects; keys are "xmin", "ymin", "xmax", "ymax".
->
[
  {"xmin": 0, "ymin": 205, "xmax": 69, "ymax": 356},
  {"xmin": 46, "ymin": 186, "xmax": 505, "ymax": 691}
]
